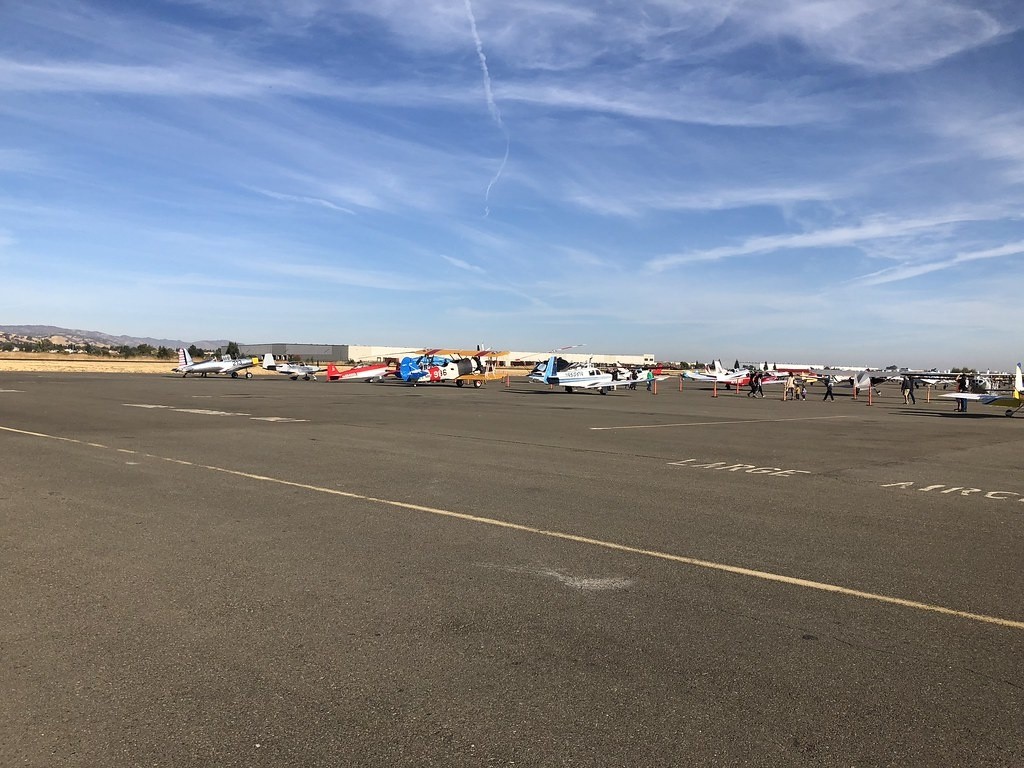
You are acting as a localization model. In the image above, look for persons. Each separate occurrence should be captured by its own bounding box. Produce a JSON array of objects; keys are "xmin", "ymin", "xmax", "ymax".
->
[
  {"xmin": 908, "ymin": 375, "xmax": 917, "ymax": 404},
  {"xmin": 801, "ymin": 385, "xmax": 807, "ymax": 400},
  {"xmin": 647, "ymin": 369, "xmax": 653, "ymax": 391},
  {"xmin": 606, "ymin": 367, "xmax": 619, "ymax": 391},
  {"xmin": 755, "ymin": 374, "xmax": 766, "ymax": 398},
  {"xmin": 784, "ymin": 371, "xmax": 795, "ymax": 400},
  {"xmin": 795, "ymin": 385, "xmax": 800, "ymax": 400},
  {"xmin": 953, "ymin": 374, "xmax": 969, "ymax": 412},
  {"xmin": 822, "ymin": 378, "xmax": 835, "ymax": 403},
  {"xmin": 747, "ymin": 372, "xmax": 758, "ymax": 399},
  {"xmin": 630, "ymin": 370, "xmax": 638, "ymax": 390},
  {"xmin": 901, "ymin": 375, "xmax": 912, "ymax": 405}
]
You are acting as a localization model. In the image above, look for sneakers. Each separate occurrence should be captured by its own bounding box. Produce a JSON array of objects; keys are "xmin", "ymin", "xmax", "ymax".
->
[
  {"xmin": 755, "ymin": 395, "xmax": 759, "ymax": 398},
  {"xmin": 747, "ymin": 393, "xmax": 750, "ymax": 397},
  {"xmin": 763, "ymin": 395, "xmax": 766, "ymax": 397}
]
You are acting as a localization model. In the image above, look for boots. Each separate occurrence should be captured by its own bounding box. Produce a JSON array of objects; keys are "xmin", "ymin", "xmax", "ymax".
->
[{"xmin": 791, "ymin": 397, "xmax": 795, "ymax": 401}]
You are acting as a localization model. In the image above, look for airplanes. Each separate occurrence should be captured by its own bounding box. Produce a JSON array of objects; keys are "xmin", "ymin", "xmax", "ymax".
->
[
  {"xmin": 525, "ymin": 356, "xmax": 660, "ymax": 395},
  {"xmin": 680, "ymin": 361, "xmax": 1016, "ymax": 396},
  {"xmin": 171, "ymin": 347, "xmax": 402, "ymax": 384}
]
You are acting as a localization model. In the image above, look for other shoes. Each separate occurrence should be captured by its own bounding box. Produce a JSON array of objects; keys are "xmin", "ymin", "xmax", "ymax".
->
[
  {"xmin": 822, "ymin": 398, "xmax": 827, "ymax": 401},
  {"xmin": 913, "ymin": 401, "xmax": 916, "ymax": 404},
  {"xmin": 830, "ymin": 399, "xmax": 835, "ymax": 402},
  {"xmin": 904, "ymin": 402, "xmax": 909, "ymax": 405},
  {"xmin": 646, "ymin": 388, "xmax": 652, "ymax": 391}
]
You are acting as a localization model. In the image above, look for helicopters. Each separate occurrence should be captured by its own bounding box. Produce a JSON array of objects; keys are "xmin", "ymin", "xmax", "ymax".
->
[
  {"xmin": 399, "ymin": 348, "xmax": 511, "ymax": 388},
  {"xmin": 938, "ymin": 362, "xmax": 1024, "ymax": 418}
]
